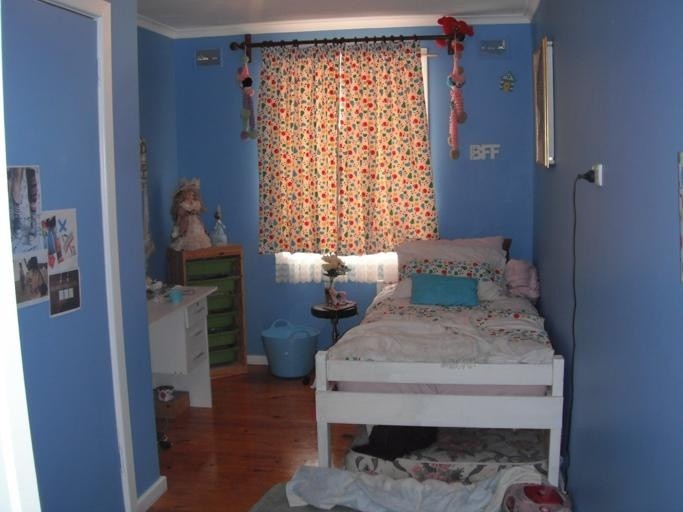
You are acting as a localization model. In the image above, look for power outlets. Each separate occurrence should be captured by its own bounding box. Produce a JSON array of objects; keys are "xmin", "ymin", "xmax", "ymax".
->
[{"xmin": 592, "ymin": 163, "xmax": 602, "ymax": 186}]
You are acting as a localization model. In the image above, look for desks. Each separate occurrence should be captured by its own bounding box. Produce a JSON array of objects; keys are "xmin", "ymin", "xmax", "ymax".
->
[
  {"xmin": 147, "ymin": 285, "xmax": 218, "ymax": 409},
  {"xmin": 303, "ymin": 301, "xmax": 358, "ymax": 390}
]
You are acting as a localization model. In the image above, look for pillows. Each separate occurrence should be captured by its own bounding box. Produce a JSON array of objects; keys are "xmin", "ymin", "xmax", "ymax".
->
[{"xmin": 394, "ymin": 236, "xmax": 508, "ymax": 306}]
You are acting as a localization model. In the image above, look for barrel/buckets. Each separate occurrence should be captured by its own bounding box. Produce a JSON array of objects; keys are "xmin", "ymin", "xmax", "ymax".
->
[{"xmin": 262, "ymin": 318, "xmax": 321, "ymax": 379}]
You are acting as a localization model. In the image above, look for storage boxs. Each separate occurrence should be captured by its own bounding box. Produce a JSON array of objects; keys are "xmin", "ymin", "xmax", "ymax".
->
[{"xmin": 154, "ymin": 388, "xmax": 190, "ymax": 418}]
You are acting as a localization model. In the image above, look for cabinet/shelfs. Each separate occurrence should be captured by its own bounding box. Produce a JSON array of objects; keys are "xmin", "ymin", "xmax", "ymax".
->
[{"xmin": 165, "ymin": 244, "xmax": 248, "ymax": 379}]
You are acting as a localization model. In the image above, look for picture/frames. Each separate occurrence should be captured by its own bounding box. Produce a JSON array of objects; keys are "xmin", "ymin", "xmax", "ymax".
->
[{"xmin": 532, "ymin": 36, "xmax": 555, "ymax": 168}]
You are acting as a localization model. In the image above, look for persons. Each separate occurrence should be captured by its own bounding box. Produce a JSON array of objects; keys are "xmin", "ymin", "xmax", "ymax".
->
[
  {"xmin": 168, "ymin": 177, "xmax": 212, "ymax": 252},
  {"xmin": 28, "ymin": 257, "xmax": 47, "ymax": 298},
  {"xmin": 207, "ymin": 211, "xmax": 227, "ymax": 246},
  {"xmin": 8, "ymin": 167, "xmax": 39, "ymax": 254}
]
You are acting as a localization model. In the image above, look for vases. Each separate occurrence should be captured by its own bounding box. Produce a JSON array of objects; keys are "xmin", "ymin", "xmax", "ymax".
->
[
  {"xmin": 324, "ymin": 282, "xmax": 334, "ymax": 306},
  {"xmin": 156, "ymin": 385, "xmax": 176, "ymax": 402}
]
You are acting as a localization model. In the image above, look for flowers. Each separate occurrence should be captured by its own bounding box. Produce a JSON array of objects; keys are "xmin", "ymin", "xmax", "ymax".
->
[{"xmin": 321, "ymin": 252, "xmax": 349, "ymax": 282}]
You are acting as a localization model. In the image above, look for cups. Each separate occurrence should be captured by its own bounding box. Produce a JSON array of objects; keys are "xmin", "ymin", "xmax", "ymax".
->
[
  {"xmin": 167, "ymin": 288, "xmax": 182, "ymax": 305},
  {"xmin": 156, "ymin": 386, "xmax": 174, "ymax": 402}
]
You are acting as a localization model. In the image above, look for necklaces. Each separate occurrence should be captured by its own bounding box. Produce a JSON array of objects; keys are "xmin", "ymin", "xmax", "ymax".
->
[{"xmin": 436, "ymin": 16, "xmax": 475, "ymax": 160}]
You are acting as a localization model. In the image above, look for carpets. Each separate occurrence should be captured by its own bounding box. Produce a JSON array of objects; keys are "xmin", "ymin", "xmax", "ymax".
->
[{"xmin": 251, "ymin": 481, "xmax": 361, "ymax": 512}]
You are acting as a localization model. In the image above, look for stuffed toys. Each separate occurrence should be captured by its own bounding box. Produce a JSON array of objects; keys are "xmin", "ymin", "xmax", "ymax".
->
[{"xmin": 235, "ymin": 56, "xmax": 257, "ymax": 140}]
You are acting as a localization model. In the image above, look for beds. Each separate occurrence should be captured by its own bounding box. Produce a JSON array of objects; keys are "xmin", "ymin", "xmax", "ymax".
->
[{"xmin": 315, "ymin": 267, "xmax": 565, "ymax": 486}]
